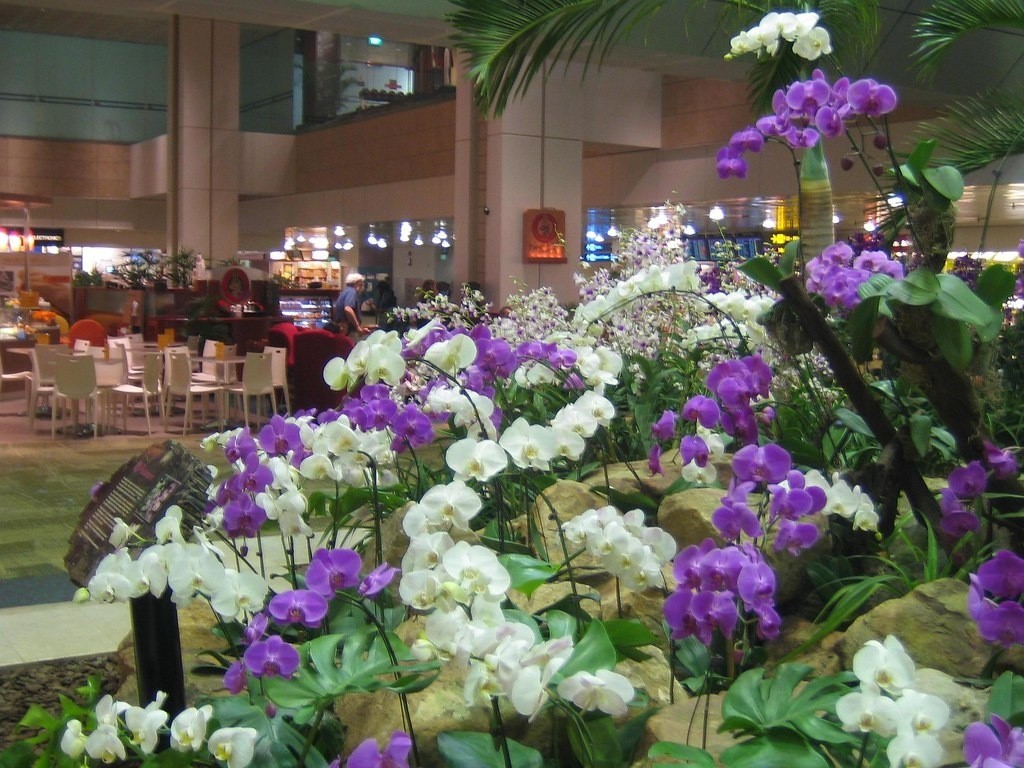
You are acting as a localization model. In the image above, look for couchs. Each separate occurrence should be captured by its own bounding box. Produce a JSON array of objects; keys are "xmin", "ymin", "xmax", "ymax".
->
[{"xmin": 267, "ymin": 322, "xmax": 356, "ymax": 422}]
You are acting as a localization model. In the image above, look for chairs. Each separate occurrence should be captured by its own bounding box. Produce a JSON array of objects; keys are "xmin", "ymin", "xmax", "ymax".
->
[{"xmin": 0, "ymin": 333, "xmax": 291, "ymax": 444}]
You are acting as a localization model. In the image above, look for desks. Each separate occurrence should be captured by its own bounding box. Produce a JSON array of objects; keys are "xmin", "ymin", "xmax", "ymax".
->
[
  {"xmin": 125, "ymin": 347, "xmax": 166, "ymax": 415},
  {"xmin": 7, "ymin": 348, "xmax": 86, "ymax": 417},
  {"xmin": 187, "ymin": 354, "xmax": 246, "ymax": 431},
  {"xmin": 132, "ymin": 342, "xmax": 188, "ymax": 403}
]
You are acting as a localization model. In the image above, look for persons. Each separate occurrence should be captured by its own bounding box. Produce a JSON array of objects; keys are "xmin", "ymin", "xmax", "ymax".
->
[{"xmin": 331, "ymin": 271, "xmax": 377, "ymax": 336}]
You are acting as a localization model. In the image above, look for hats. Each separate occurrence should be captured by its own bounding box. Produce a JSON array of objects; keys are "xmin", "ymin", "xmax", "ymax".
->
[{"xmin": 346, "ymin": 273, "xmax": 365, "ymax": 284}]
